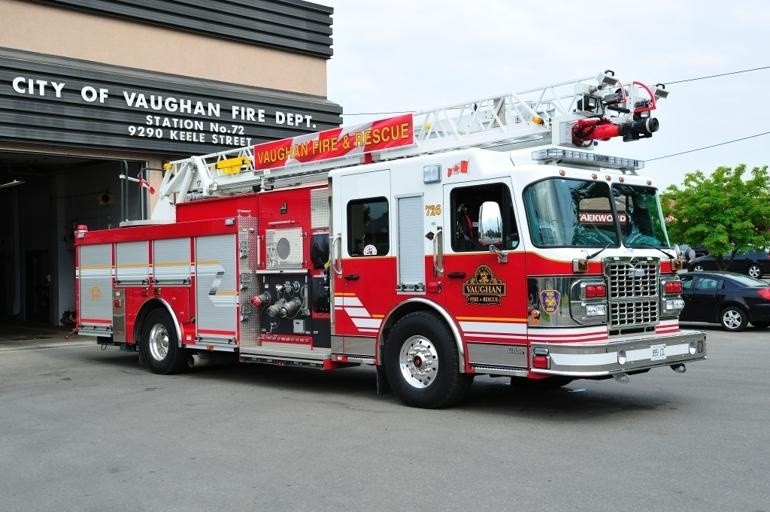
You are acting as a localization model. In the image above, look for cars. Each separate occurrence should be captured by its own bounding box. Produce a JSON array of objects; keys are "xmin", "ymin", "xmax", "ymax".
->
[
  {"xmin": 677, "ymin": 245, "xmax": 707, "ymax": 269},
  {"xmin": 687, "ymin": 244, "xmax": 770, "ymax": 279},
  {"xmin": 678, "ymin": 272, "xmax": 770, "ymax": 332}
]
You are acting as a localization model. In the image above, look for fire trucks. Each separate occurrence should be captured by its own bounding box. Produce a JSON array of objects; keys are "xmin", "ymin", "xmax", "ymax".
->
[{"xmin": 73, "ymin": 68, "xmax": 707, "ymax": 407}]
